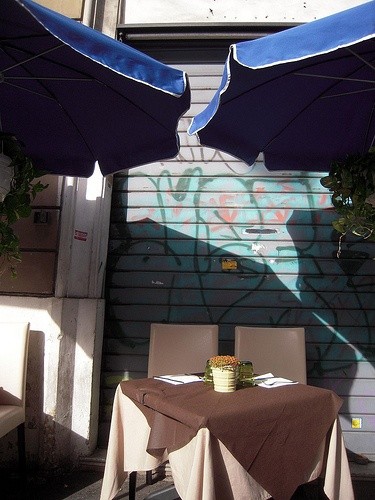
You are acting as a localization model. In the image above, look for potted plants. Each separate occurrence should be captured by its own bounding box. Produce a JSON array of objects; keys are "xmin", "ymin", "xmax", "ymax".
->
[{"xmin": 0, "ymin": 131, "xmax": 50, "ymax": 280}]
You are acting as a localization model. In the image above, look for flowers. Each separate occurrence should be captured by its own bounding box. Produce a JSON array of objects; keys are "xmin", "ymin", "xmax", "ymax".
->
[
  {"xmin": 209, "ymin": 356, "xmax": 240, "ymax": 367},
  {"xmin": 320, "ymin": 147, "xmax": 375, "ymax": 259}
]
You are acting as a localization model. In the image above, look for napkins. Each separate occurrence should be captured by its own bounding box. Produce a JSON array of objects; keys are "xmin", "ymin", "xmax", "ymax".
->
[
  {"xmin": 154, "ymin": 374, "xmax": 212, "ymax": 385},
  {"xmin": 241, "ymin": 372, "xmax": 299, "ymax": 388}
]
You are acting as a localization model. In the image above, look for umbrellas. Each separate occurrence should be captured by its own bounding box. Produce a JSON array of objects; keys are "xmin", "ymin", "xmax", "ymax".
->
[
  {"xmin": 186, "ymin": 0, "xmax": 375, "ymax": 172},
  {"xmin": 0, "ymin": 0, "xmax": 192, "ymax": 179}
]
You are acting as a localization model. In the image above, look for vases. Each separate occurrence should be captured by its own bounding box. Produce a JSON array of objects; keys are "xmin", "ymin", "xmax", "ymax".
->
[{"xmin": 209, "ymin": 367, "xmax": 241, "ymax": 393}]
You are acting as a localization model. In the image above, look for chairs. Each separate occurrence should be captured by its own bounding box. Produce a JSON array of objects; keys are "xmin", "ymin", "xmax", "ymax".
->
[
  {"xmin": 234, "ymin": 326, "xmax": 307, "ymax": 387},
  {"xmin": 0, "ymin": 322, "xmax": 30, "ymax": 468},
  {"xmin": 145, "ymin": 324, "xmax": 218, "ymax": 484}
]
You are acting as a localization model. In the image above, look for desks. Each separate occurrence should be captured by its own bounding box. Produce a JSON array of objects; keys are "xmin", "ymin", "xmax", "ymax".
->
[{"xmin": 100, "ymin": 373, "xmax": 355, "ymax": 500}]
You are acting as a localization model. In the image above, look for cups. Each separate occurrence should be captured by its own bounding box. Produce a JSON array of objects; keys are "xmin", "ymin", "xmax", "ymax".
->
[
  {"xmin": 235, "ymin": 361, "xmax": 255, "ymax": 387},
  {"xmin": 202, "ymin": 360, "xmax": 214, "ymax": 384}
]
